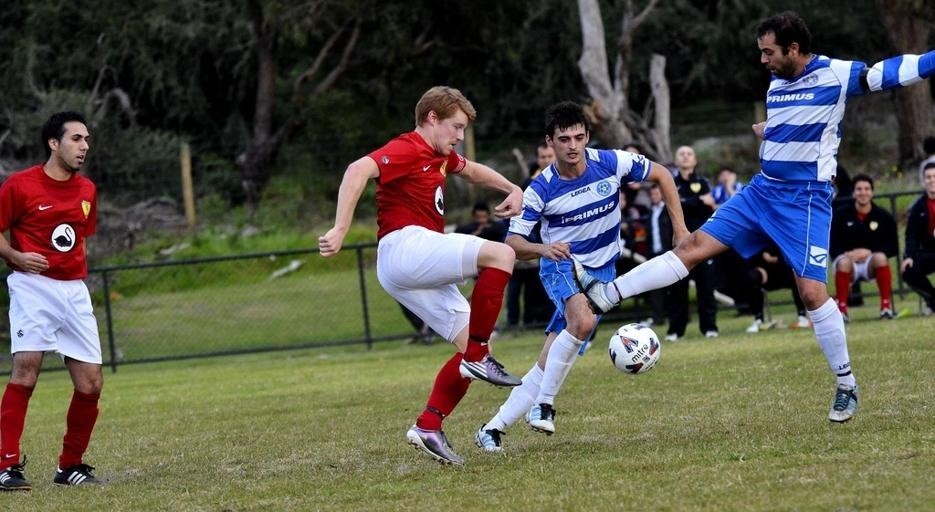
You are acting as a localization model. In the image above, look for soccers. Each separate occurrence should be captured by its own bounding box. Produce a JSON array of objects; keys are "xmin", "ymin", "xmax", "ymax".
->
[{"xmin": 607, "ymin": 326, "xmax": 659, "ymax": 377}]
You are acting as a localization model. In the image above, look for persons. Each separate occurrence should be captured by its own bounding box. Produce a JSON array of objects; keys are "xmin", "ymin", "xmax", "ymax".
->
[
  {"xmin": 1, "ymin": 110, "xmax": 126, "ymax": 493},
  {"xmin": 570, "ymin": 13, "xmax": 935, "ymax": 425},
  {"xmin": 475, "ymin": 98, "xmax": 693, "ymax": 452},
  {"xmin": 319, "ymin": 85, "xmax": 524, "ymax": 470},
  {"xmin": 400, "ymin": 142, "xmax": 935, "ymax": 348}
]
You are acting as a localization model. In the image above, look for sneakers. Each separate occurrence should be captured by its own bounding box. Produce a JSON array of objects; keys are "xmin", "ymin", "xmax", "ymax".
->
[
  {"xmin": 407, "ymin": 420, "xmax": 465, "ymax": 466},
  {"xmin": 525, "ymin": 403, "xmax": 556, "ymax": 437},
  {"xmin": 744, "ymin": 318, "xmax": 763, "ymax": 335},
  {"xmin": 881, "ymin": 307, "xmax": 892, "ymax": 318},
  {"xmin": 664, "ymin": 334, "xmax": 679, "ymax": 341},
  {"xmin": 0, "ymin": 467, "xmax": 31, "ymax": 490},
  {"xmin": 54, "ymin": 463, "xmax": 98, "ymax": 486},
  {"xmin": 459, "ymin": 353, "xmax": 521, "ymax": 386},
  {"xmin": 573, "ymin": 256, "xmax": 619, "ymax": 314},
  {"xmin": 473, "ymin": 423, "xmax": 504, "ymax": 455},
  {"xmin": 706, "ymin": 333, "xmax": 718, "ymax": 337},
  {"xmin": 712, "ymin": 288, "xmax": 740, "ymax": 308},
  {"xmin": 788, "ymin": 317, "xmax": 813, "ymax": 329},
  {"xmin": 827, "ymin": 387, "xmax": 857, "ymax": 424}
]
